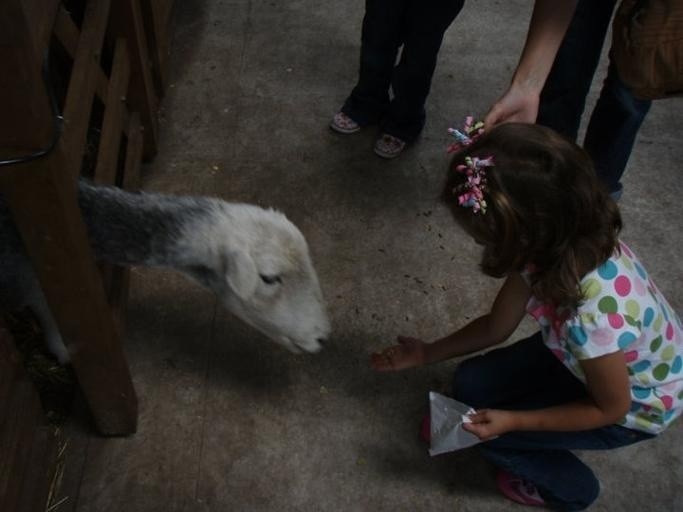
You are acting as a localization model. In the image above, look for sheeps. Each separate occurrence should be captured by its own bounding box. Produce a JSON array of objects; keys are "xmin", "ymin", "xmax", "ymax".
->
[{"xmin": 0, "ymin": 139, "xmax": 332, "ymax": 362}]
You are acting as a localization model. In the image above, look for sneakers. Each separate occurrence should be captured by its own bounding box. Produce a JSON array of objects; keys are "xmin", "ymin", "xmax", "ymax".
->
[
  {"xmin": 493, "ymin": 463, "xmax": 547, "ymax": 507},
  {"xmin": 329, "ymin": 111, "xmax": 360, "ymax": 134},
  {"xmin": 373, "ymin": 133, "xmax": 406, "ymax": 159}
]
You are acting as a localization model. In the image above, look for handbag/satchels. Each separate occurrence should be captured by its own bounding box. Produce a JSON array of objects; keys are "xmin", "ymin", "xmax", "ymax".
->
[{"xmin": 607, "ymin": 0, "xmax": 683, "ymax": 101}]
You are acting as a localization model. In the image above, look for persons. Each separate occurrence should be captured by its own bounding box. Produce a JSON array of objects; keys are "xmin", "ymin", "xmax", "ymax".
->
[
  {"xmin": 368, "ymin": 122, "xmax": 683, "ymax": 508},
  {"xmin": 482, "ymin": 0, "xmax": 656, "ymax": 202},
  {"xmin": 329, "ymin": 3, "xmax": 462, "ymax": 158}
]
